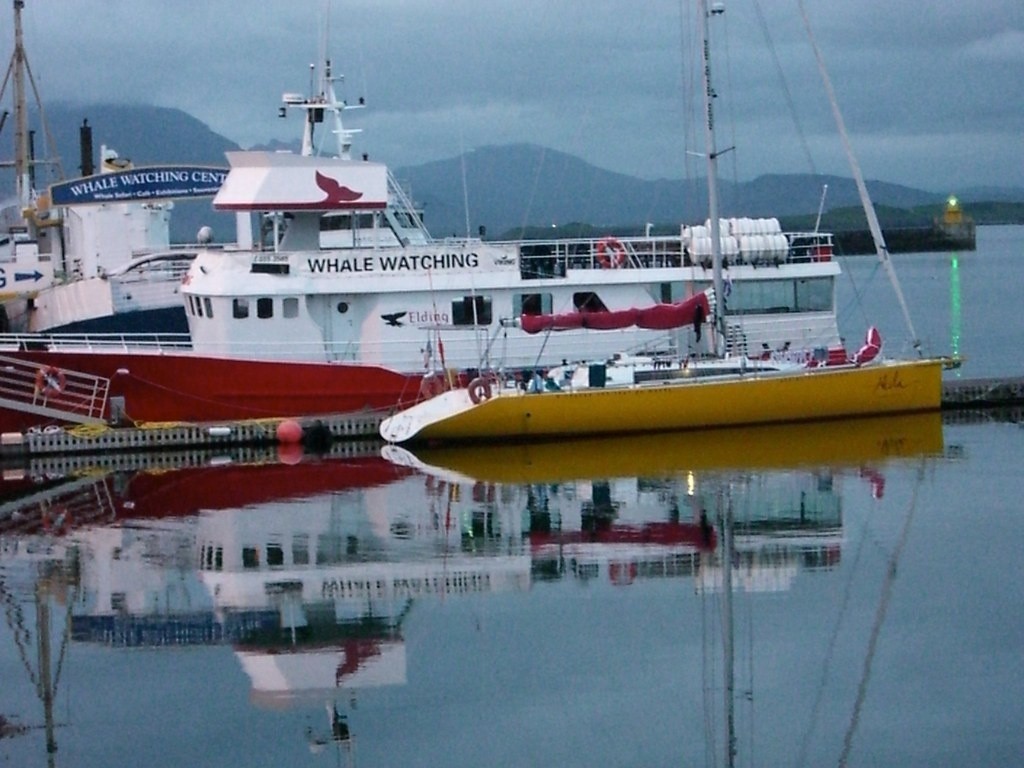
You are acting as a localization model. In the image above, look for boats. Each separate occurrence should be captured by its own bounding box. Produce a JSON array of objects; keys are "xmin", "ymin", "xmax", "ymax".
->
[
  {"xmin": 197, "ymin": 464, "xmax": 842, "ymax": 768},
  {"xmin": 176, "ymin": 0, "xmax": 846, "ymax": 388},
  {"xmin": 0, "ymin": 0, "xmax": 296, "ymax": 345}
]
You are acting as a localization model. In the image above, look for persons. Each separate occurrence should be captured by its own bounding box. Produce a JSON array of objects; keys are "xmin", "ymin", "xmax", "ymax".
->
[{"xmin": 514, "ymin": 359, "xmax": 588, "ymax": 393}]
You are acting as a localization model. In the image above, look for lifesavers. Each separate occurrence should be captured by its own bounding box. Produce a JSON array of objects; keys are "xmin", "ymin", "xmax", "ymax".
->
[
  {"xmin": 469, "ymin": 377, "xmax": 490, "ymax": 404},
  {"xmin": 420, "ymin": 376, "xmax": 443, "ymax": 399},
  {"xmin": 597, "ymin": 236, "xmax": 626, "ymax": 268}
]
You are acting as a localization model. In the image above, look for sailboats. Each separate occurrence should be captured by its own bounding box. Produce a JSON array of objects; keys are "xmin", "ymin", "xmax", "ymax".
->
[
  {"xmin": 381, "ymin": 409, "xmax": 943, "ymax": 768},
  {"xmin": 376, "ymin": 0, "xmax": 966, "ymax": 444}
]
